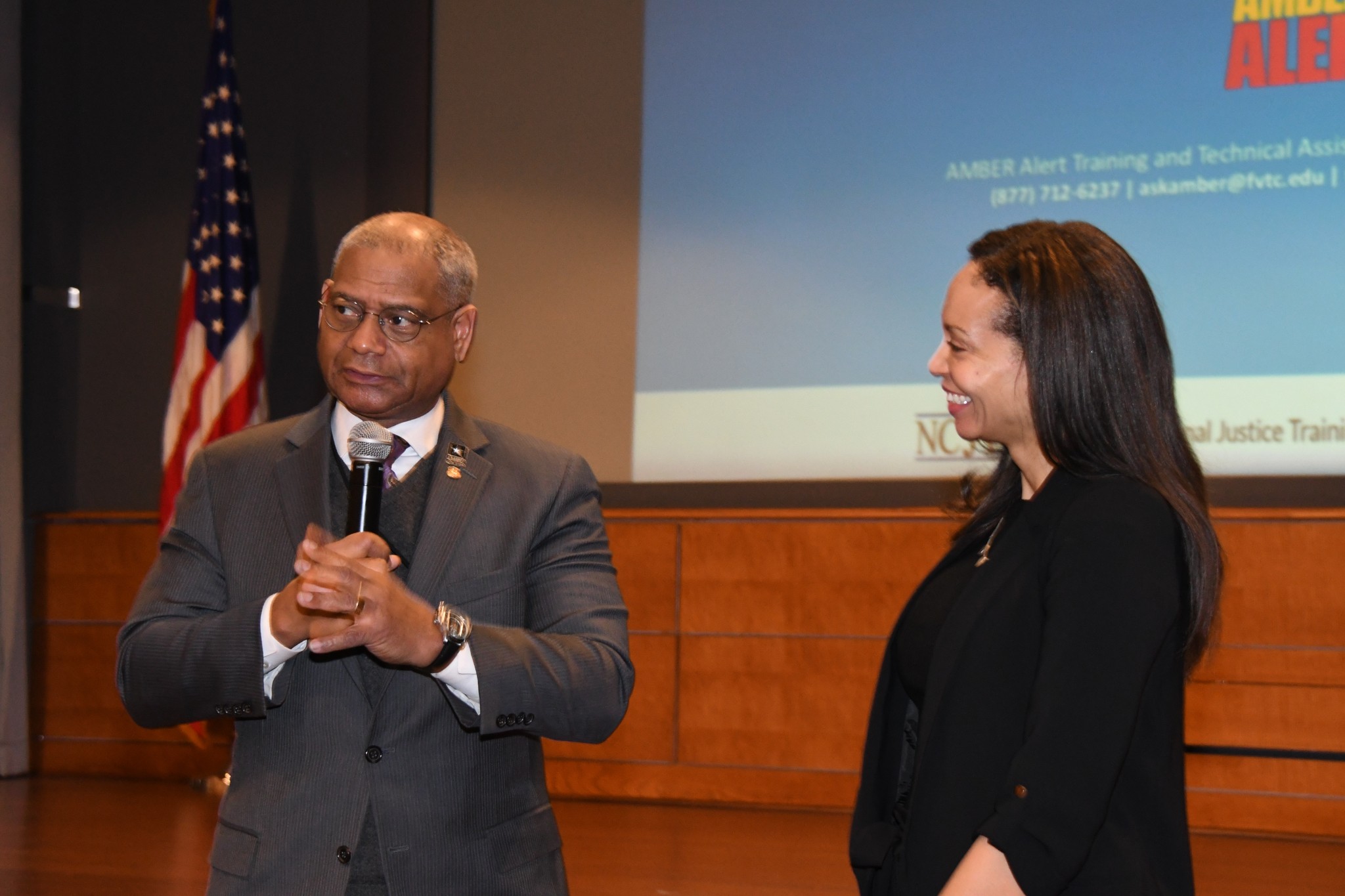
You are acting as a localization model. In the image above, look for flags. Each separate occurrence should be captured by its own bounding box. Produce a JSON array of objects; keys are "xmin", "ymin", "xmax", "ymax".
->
[{"xmin": 153, "ymin": 7, "xmax": 270, "ymax": 759}]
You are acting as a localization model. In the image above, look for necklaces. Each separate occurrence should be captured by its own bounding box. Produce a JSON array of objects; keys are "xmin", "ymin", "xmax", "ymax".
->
[{"xmin": 973, "ymin": 505, "xmax": 1007, "ymax": 567}]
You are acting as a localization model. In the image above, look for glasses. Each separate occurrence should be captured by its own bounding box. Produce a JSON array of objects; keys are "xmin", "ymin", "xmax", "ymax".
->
[{"xmin": 318, "ymin": 286, "xmax": 465, "ymax": 343}]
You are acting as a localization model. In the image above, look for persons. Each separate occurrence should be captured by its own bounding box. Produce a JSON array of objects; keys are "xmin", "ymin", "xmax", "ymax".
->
[
  {"xmin": 847, "ymin": 216, "xmax": 1228, "ymax": 895},
  {"xmin": 113, "ymin": 209, "xmax": 638, "ymax": 896}
]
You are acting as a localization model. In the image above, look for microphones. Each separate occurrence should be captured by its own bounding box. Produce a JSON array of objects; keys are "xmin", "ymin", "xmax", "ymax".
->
[{"xmin": 346, "ymin": 421, "xmax": 392, "ymax": 538}]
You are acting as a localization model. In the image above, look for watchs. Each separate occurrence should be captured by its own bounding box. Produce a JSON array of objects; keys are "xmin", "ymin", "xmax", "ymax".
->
[{"xmin": 422, "ymin": 596, "xmax": 474, "ymax": 674}]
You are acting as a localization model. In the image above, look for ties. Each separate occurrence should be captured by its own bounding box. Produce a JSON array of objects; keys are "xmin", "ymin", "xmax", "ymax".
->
[{"xmin": 380, "ymin": 434, "xmax": 411, "ymax": 496}]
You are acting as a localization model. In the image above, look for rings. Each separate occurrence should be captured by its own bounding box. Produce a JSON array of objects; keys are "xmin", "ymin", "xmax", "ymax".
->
[{"xmin": 347, "ymin": 590, "xmax": 368, "ymax": 616}]
